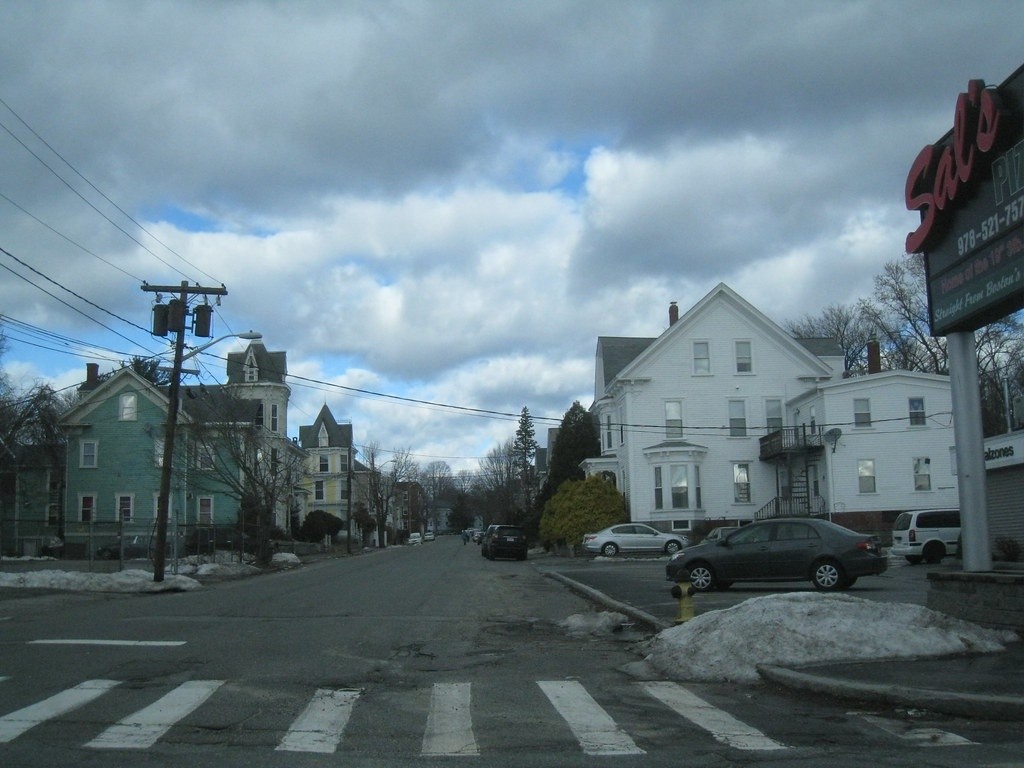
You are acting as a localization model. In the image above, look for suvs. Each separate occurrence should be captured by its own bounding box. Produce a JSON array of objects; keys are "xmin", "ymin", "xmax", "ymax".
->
[
  {"xmin": 890, "ymin": 508, "xmax": 962, "ymax": 565},
  {"xmin": 480, "ymin": 523, "xmax": 515, "ymax": 557},
  {"xmin": 486, "ymin": 525, "xmax": 530, "ymax": 561}
]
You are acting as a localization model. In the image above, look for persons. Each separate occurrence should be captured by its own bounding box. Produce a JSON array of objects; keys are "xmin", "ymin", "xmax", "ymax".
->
[{"xmin": 462, "ymin": 529, "xmax": 469, "ymax": 545}]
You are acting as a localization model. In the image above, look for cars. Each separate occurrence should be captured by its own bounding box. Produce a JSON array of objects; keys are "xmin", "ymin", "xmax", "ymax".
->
[
  {"xmin": 42, "ymin": 540, "xmax": 141, "ymax": 559},
  {"xmin": 408, "ymin": 532, "xmax": 423, "ymax": 545},
  {"xmin": 582, "ymin": 522, "xmax": 689, "ymax": 558},
  {"xmin": 665, "ymin": 518, "xmax": 888, "ymax": 592},
  {"xmin": 176, "ymin": 525, "xmax": 249, "ymax": 557},
  {"xmin": 462, "ymin": 528, "xmax": 486, "ymax": 545},
  {"xmin": 424, "ymin": 532, "xmax": 435, "ymax": 541},
  {"xmin": 698, "ymin": 526, "xmax": 741, "ymax": 544}
]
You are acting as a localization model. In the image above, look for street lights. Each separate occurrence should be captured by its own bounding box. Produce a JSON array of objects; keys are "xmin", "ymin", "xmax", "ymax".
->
[{"xmin": 151, "ymin": 328, "xmax": 264, "ymax": 584}]
